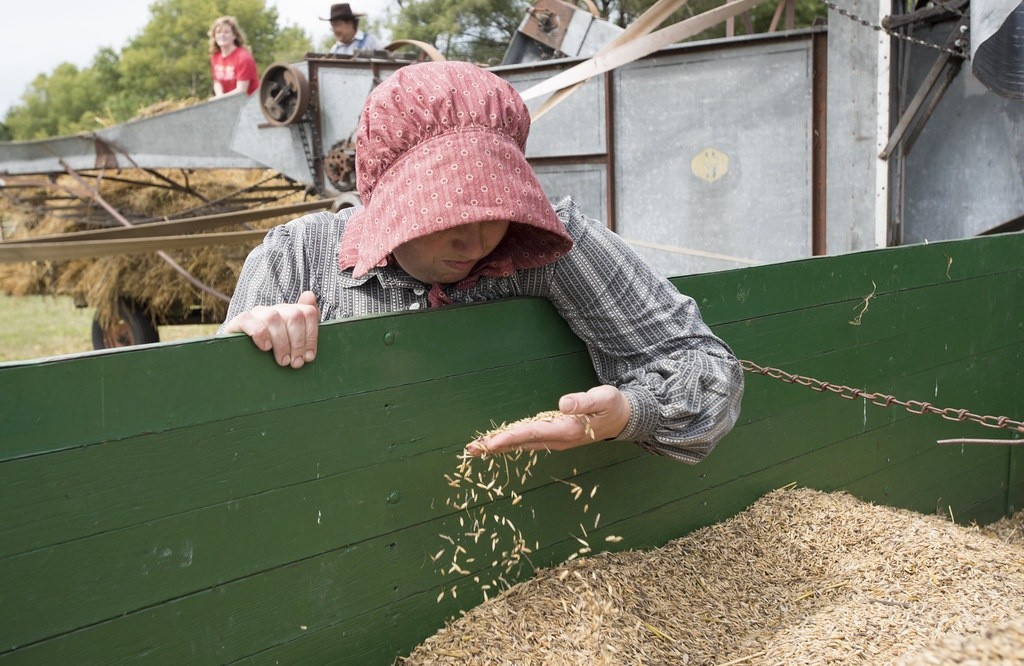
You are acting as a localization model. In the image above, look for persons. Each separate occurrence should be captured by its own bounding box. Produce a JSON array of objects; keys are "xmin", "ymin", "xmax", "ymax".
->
[
  {"xmin": 216, "ymin": 60, "xmax": 743, "ymax": 466},
  {"xmin": 320, "ymin": 2, "xmax": 382, "ymax": 55},
  {"xmin": 207, "ymin": 17, "xmax": 259, "ymax": 99}
]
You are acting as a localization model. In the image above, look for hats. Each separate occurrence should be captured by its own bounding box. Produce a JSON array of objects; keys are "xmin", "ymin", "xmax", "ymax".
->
[
  {"xmin": 338, "ymin": 59, "xmax": 575, "ymax": 280},
  {"xmin": 318, "ymin": 3, "xmax": 367, "ymax": 22}
]
